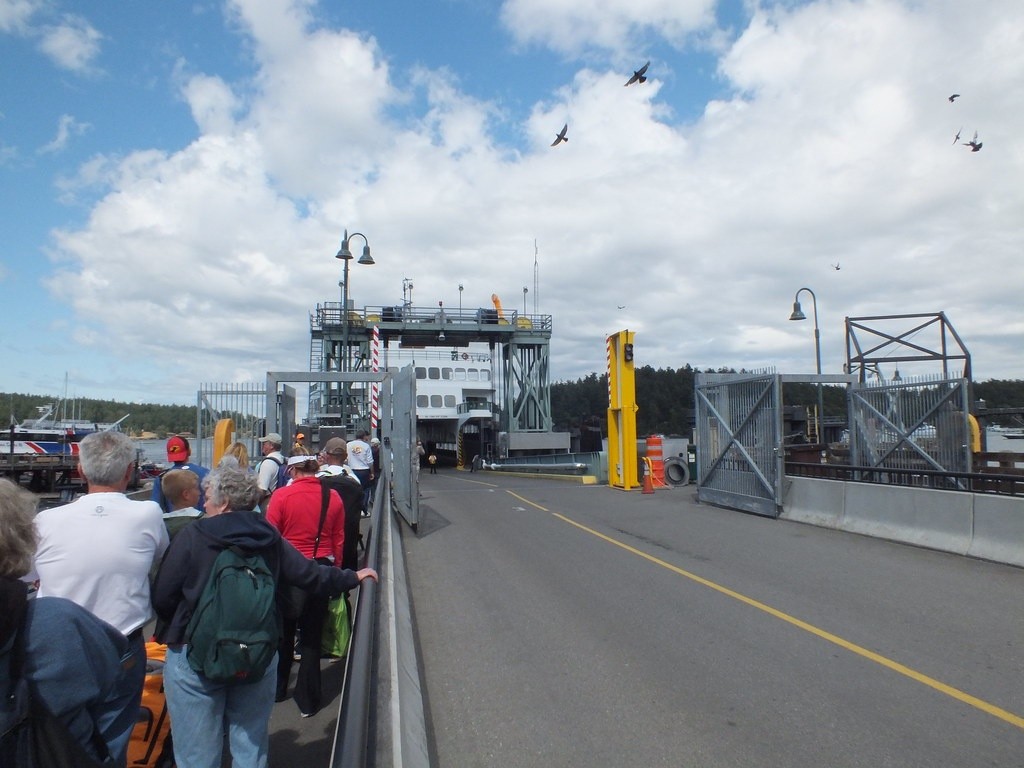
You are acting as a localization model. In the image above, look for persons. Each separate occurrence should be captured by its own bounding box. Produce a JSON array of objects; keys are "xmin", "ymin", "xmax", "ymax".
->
[
  {"xmin": 416, "ymin": 435, "xmax": 426, "ymax": 495},
  {"xmin": 222, "ymin": 442, "xmax": 247, "ymax": 469},
  {"xmin": 0, "ymin": 432, "xmax": 210, "ymax": 768},
  {"xmin": 256, "ymin": 429, "xmax": 394, "ymax": 718},
  {"xmin": 429, "ymin": 452, "xmax": 437, "ymax": 474},
  {"xmin": 152, "ymin": 458, "xmax": 380, "ymax": 768},
  {"xmin": 470, "ymin": 454, "xmax": 480, "ymax": 472}
]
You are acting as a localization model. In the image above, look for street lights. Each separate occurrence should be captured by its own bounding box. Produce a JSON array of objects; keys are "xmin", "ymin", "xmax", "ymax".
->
[
  {"xmin": 788, "ymin": 287, "xmax": 825, "ymax": 444},
  {"xmin": 335, "ymin": 228, "xmax": 375, "ymax": 426}
]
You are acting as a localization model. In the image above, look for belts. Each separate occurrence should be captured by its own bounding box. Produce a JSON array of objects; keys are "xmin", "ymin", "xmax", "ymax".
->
[{"xmin": 128, "ymin": 627, "xmax": 143, "ymax": 641}]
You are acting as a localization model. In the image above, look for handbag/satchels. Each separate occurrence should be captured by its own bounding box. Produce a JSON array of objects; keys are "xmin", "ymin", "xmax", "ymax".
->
[{"xmin": 320, "ymin": 591, "xmax": 349, "ymax": 657}]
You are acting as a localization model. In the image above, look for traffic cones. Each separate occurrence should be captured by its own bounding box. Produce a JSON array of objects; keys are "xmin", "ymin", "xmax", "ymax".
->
[{"xmin": 641, "ymin": 434, "xmax": 664, "ymax": 494}]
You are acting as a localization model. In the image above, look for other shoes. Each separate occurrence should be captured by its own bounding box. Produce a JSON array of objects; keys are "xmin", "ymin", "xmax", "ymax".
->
[
  {"xmin": 292, "ymin": 639, "xmax": 301, "ymax": 660},
  {"xmin": 301, "ymin": 695, "xmax": 323, "ymax": 718},
  {"xmin": 361, "ymin": 512, "xmax": 371, "ymax": 518}
]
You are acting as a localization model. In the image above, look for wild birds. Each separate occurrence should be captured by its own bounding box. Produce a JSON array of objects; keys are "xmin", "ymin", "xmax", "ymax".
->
[
  {"xmin": 949, "ymin": 94, "xmax": 982, "ymax": 152},
  {"xmin": 550, "ymin": 123, "xmax": 569, "ymax": 146},
  {"xmin": 624, "ymin": 60, "xmax": 650, "ymax": 86},
  {"xmin": 832, "ymin": 261, "xmax": 840, "ymax": 271}
]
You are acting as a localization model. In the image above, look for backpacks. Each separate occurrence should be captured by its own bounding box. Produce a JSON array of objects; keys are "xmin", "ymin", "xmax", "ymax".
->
[
  {"xmin": 0, "ymin": 601, "xmax": 100, "ymax": 768},
  {"xmin": 258, "ymin": 457, "xmax": 293, "ymax": 494},
  {"xmin": 183, "ymin": 545, "xmax": 283, "ymax": 685}
]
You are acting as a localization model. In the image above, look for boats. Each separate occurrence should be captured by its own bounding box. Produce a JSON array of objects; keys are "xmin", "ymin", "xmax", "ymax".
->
[{"xmin": 1, "ymin": 402, "xmax": 169, "ymax": 479}]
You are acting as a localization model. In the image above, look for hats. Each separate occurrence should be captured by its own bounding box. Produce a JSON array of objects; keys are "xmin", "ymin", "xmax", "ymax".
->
[
  {"xmin": 296, "ymin": 433, "xmax": 305, "ymax": 439},
  {"xmin": 325, "ymin": 437, "xmax": 347, "ymax": 454},
  {"xmin": 166, "ymin": 435, "xmax": 189, "ymax": 462},
  {"xmin": 258, "ymin": 433, "xmax": 283, "ymax": 445},
  {"xmin": 364, "ymin": 431, "xmax": 370, "ymax": 435}
]
